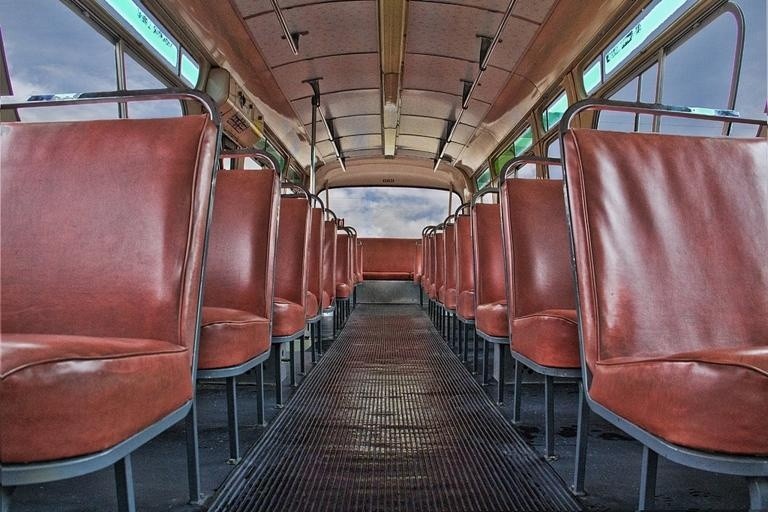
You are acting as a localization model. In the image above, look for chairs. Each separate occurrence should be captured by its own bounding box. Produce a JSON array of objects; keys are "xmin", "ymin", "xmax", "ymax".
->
[
  {"xmin": 497, "ymin": 155, "xmax": 583, "ymax": 467},
  {"xmin": 417, "ymin": 222, "xmax": 448, "ymax": 341},
  {"xmin": 308, "ymin": 192, "xmax": 325, "ymax": 357},
  {"xmin": 555, "ymin": 99, "xmax": 768, "ymax": 508},
  {"xmin": 441, "ymin": 213, "xmax": 455, "ymax": 344},
  {"xmin": 453, "ymin": 201, "xmax": 475, "ymax": 366},
  {"xmin": 1, "ymin": 88, "xmax": 225, "ymax": 506},
  {"xmin": 470, "ymin": 185, "xmax": 511, "ymax": 389},
  {"xmin": 272, "ymin": 177, "xmax": 314, "ymax": 410},
  {"xmin": 197, "ymin": 141, "xmax": 286, "ymax": 468},
  {"xmin": 336, "ymin": 225, "xmax": 358, "ymax": 328},
  {"xmin": 323, "ymin": 206, "xmax": 340, "ymax": 344}
]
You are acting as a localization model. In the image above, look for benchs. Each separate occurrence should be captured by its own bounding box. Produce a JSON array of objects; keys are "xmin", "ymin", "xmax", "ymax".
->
[{"xmin": 360, "ymin": 238, "xmax": 420, "ymax": 280}]
